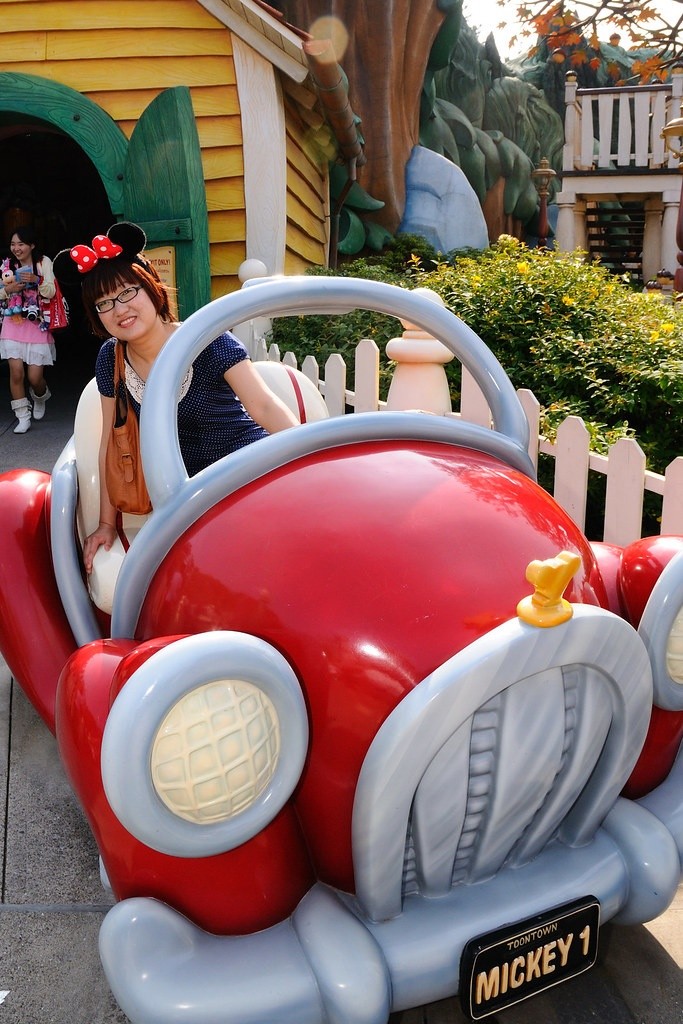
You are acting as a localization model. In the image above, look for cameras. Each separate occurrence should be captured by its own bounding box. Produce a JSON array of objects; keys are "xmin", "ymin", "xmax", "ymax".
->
[{"xmin": 22, "ymin": 305, "xmax": 40, "ymax": 321}]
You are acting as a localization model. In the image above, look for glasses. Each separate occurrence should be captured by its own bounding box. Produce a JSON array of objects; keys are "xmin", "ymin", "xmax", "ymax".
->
[{"xmin": 95, "ymin": 286, "xmax": 143, "ymax": 313}]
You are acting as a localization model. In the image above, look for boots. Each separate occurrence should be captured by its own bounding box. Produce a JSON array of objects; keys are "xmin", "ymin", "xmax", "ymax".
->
[
  {"xmin": 29, "ymin": 383, "xmax": 51, "ymax": 420},
  {"xmin": 11, "ymin": 397, "xmax": 32, "ymax": 434}
]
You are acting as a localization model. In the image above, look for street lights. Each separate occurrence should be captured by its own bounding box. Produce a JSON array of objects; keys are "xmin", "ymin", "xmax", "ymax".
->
[
  {"xmin": 532, "ymin": 158, "xmax": 555, "ymax": 249},
  {"xmin": 658, "ymin": 101, "xmax": 683, "ymax": 293}
]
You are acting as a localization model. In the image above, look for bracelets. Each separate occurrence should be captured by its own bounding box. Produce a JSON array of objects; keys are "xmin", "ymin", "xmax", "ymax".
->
[{"xmin": 35, "ymin": 275, "xmax": 43, "ymax": 286}]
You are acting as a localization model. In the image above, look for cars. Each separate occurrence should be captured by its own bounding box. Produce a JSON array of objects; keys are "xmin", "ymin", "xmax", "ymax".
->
[{"xmin": 2, "ymin": 277, "xmax": 682, "ymax": 1022}]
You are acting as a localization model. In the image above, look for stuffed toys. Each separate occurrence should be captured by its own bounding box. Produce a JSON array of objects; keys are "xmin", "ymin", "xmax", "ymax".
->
[{"xmin": 0, "ymin": 259, "xmax": 38, "ymax": 322}]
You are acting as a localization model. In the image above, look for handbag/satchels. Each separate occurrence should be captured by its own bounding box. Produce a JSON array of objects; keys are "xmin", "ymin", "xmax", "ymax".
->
[
  {"xmin": 105, "ymin": 341, "xmax": 151, "ymax": 514},
  {"xmin": 39, "ymin": 278, "xmax": 69, "ymax": 332}
]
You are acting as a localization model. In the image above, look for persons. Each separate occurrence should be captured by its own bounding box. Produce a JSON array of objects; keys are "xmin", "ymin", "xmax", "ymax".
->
[
  {"xmin": 0, "ymin": 230, "xmax": 55, "ymax": 433},
  {"xmin": 52, "ymin": 221, "xmax": 302, "ymax": 573}
]
